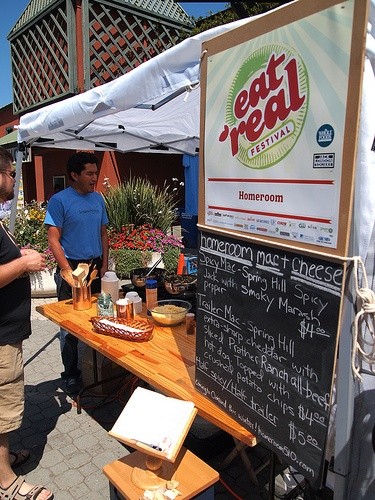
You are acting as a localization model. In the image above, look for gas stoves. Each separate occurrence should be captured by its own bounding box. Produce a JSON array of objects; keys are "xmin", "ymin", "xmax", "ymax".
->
[{"xmin": 121, "ymin": 284, "xmax": 197, "ymax": 303}]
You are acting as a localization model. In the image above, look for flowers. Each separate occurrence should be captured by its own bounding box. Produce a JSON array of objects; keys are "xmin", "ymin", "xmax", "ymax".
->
[{"xmin": 1, "ymin": 173, "xmax": 186, "ymax": 279}]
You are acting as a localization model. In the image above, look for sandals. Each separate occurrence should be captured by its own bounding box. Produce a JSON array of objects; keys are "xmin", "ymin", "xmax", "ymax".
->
[
  {"xmin": 0, "ymin": 474, "xmax": 54, "ymax": 500},
  {"xmin": 9, "ymin": 449, "xmax": 30, "ymax": 467}
]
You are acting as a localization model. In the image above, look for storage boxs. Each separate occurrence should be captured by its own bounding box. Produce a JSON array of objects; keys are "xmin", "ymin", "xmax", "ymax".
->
[{"xmin": 89, "ymin": 315, "xmax": 155, "ymax": 343}]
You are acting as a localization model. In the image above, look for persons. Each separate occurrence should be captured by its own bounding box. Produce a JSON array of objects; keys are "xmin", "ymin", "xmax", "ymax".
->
[
  {"xmin": 44, "ymin": 151, "xmax": 110, "ymax": 392},
  {"xmin": 0, "ymin": 145, "xmax": 54, "ymax": 500}
]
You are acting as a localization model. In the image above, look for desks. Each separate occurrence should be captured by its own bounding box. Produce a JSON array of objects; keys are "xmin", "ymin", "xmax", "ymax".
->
[{"xmin": 34, "ymin": 291, "xmax": 272, "ymax": 485}]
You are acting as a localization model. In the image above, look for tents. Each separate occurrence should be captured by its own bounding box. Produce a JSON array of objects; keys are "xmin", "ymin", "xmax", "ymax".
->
[{"xmin": 8, "ymin": 0, "xmax": 375, "ymax": 499}]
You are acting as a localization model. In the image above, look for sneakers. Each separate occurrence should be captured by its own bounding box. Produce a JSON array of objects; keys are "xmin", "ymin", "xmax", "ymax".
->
[{"xmin": 65, "ymin": 376, "xmax": 80, "ymax": 394}]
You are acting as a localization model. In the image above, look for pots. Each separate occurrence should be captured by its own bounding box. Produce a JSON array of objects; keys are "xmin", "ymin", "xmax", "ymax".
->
[
  {"xmin": 164, "ymin": 274, "xmax": 197, "ymax": 294},
  {"xmin": 127, "ymin": 267, "xmax": 166, "ymax": 288}
]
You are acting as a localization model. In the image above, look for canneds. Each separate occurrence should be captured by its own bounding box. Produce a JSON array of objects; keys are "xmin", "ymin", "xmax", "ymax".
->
[
  {"xmin": 146, "ymin": 280, "xmax": 158, "ymax": 316},
  {"xmin": 185, "ymin": 313, "xmax": 195, "ymax": 334}
]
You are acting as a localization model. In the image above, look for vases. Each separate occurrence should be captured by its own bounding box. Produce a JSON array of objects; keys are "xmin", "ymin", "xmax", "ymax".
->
[{"xmin": 30, "ymin": 251, "xmax": 164, "ymax": 297}]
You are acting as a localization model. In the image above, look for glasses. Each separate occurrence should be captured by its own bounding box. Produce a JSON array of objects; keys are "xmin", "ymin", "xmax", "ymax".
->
[{"xmin": 0, "ymin": 169, "xmax": 17, "ymax": 178}]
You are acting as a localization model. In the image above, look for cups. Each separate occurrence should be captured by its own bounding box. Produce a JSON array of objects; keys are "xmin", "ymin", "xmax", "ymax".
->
[
  {"xmin": 146, "ymin": 288, "xmax": 157, "ymax": 316},
  {"xmin": 186, "ymin": 313, "xmax": 195, "ymax": 335},
  {"xmin": 116, "ymin": 299, "xmax": 134, "ymax": 320},
  {"xmin": 72, "ymin": 285, "xmax": 92, "ymax": 311}
]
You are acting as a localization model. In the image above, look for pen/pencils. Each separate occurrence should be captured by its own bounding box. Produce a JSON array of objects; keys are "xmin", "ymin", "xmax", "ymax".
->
[{"xmin": 131, "ymin": 437, "xmax": 163, "ymax": 452}]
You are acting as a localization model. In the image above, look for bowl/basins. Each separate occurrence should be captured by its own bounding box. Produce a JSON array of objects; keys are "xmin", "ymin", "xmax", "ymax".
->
[{"xmin": 148, "ymin": 299, "xmax": 192, "ymax": 324}]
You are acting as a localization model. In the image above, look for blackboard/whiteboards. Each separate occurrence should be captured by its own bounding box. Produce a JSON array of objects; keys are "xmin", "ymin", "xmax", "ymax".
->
[{"xmin": 193, "ymin": 230, "xmax": 346, "ymax": 491}]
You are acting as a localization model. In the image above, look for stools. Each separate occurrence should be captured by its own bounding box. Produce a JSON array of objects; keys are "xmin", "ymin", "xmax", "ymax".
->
[{"xmin": 101, "ymin": 443, "xmax": 220, "ymax": 500}]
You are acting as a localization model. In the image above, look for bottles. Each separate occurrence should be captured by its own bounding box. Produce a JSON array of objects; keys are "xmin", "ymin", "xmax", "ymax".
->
[
  {"xmin": 97, "ymin": 293, "xmax": 114, "ymax": 318},
  {"xmin": 177, "ymin": 254, "xmax": 187, "ymax": 275}
]
